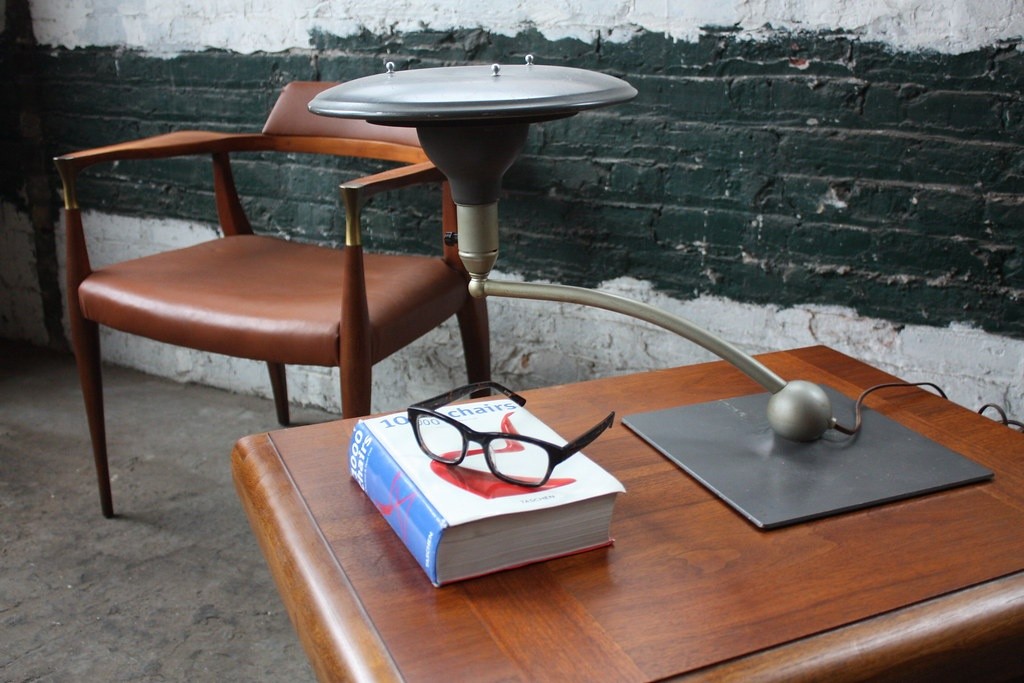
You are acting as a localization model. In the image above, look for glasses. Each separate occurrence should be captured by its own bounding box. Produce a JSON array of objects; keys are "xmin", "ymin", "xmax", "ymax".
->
[{"xmin": 407, "ymin": 381, "xmax": 614, "ymax": 487}]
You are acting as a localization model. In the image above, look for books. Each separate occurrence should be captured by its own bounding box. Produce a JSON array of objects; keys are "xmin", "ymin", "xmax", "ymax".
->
[{"xmin": 348, "ymin": 399, "xmax": 626, "ymax": 589}]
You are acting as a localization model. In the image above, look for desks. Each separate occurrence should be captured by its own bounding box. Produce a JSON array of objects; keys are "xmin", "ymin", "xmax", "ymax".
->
[{"xmin": 230, "ymin": 345, "xmax": 1024, "ymax": 683}]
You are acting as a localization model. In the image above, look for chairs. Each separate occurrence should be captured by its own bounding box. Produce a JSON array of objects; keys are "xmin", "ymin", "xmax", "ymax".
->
[{"xmin": 52, "ymin": 79, "xmax": 494, "ymax": 521}]
